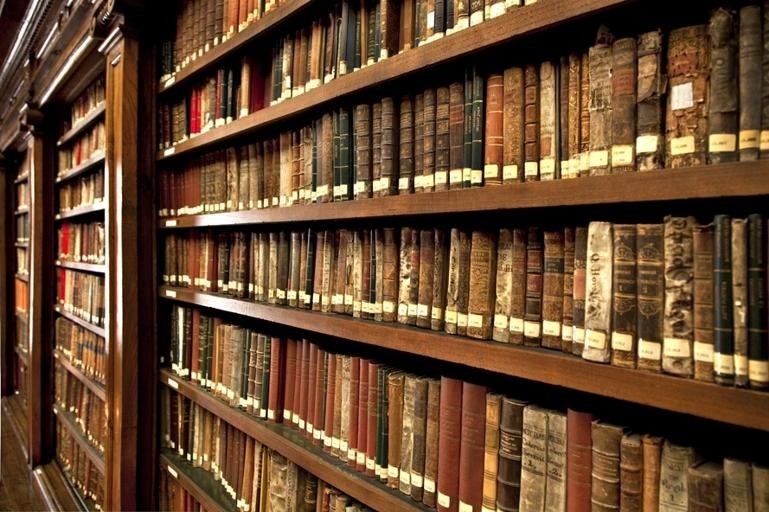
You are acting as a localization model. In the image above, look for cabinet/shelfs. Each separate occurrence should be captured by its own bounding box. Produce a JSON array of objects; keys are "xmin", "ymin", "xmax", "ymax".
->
[
  {"xmin": 112, "ymin": 0, "xmax": 768, "ymax": 512},
  {"xmin": 26, "ymin": 0, "xmax": 111, "ymax": 511},
  {"xmin": 0, "ymin": 11, "xmax": 32, "ymax": 476}
]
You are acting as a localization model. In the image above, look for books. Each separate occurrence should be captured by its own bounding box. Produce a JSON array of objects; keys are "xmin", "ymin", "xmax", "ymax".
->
[
  {"xmin": 0, "ymin": 45, "xmax": 108, "ymax": 510},
  {"xmin": 156, "ymin": 3, "xmax": 769, "ymax": 511}
]
